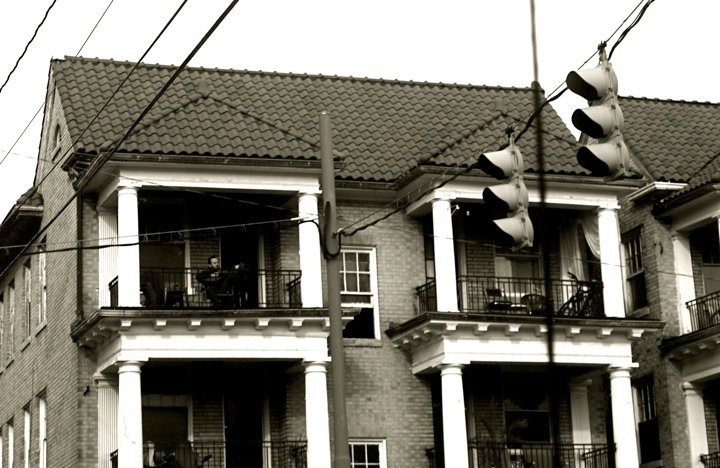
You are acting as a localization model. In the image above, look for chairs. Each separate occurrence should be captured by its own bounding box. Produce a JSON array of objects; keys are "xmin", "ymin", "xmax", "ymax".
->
[
  {"xmin": 142, "ymin": 270, "xmax": 165, "ymax": 307},
  {"xmin": 521, "ymin": 294, "xmax": 546, "ymax": 316},
  {"xmin": 486, "ymin": 287, "xmax": 530, "ymax": 313},
  {"xmin": 174, "ymin": 443, "xmax": 212, "ymax": 468}
]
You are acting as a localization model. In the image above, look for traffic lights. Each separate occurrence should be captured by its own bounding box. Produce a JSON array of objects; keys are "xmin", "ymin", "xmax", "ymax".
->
[
  {"xmin": 477, "ymin": 124, "xmax": 535, "ymax": 254},
  {"xmin": 566, "ymin": 49, "xmax": 627, "ymax": 183}
]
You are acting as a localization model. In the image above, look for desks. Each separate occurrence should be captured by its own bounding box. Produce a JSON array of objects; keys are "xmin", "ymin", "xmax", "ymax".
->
[
  {"xmin": 164, "ymin": 286, "xmax": 189, "ymax": 307},
  {"xmin": 216, "ymin": 292, "xmax": 243, "ymax": 308}
]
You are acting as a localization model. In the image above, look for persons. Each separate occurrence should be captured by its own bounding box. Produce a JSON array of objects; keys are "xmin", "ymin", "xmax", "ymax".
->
[{"xmin": 197, "ymin": 256, "xmax": 243, "ymax": 300}]
